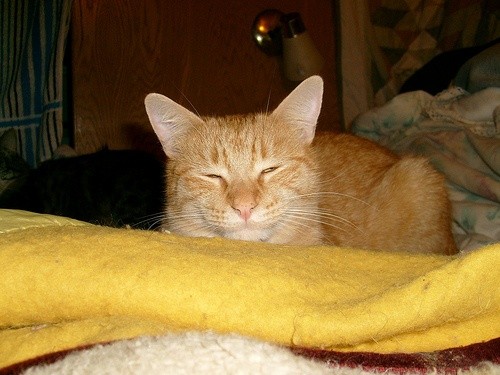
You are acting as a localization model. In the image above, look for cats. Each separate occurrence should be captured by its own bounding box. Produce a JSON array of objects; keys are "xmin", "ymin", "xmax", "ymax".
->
[{"xmin": 132, "ymin": 74, "xmax": 459, "ymax": 256}]
